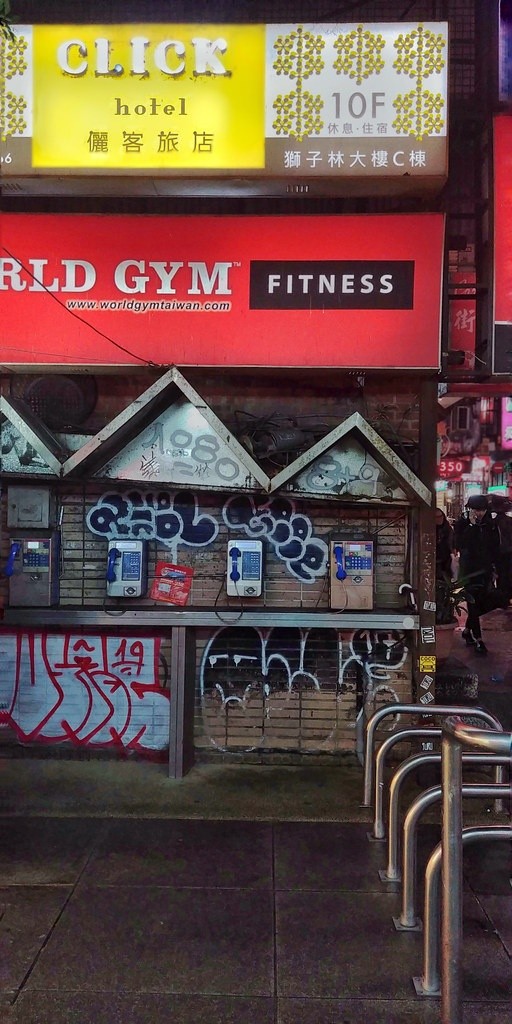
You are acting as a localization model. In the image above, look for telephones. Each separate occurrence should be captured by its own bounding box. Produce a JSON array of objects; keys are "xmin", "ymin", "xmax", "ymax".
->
[
  {"xmin": 226, "ymin": 539, "xmax": 266, "ymax": 597},
  {"xmin": 106, "ymin": 538, "xmax": 148, "ymax": 597},
  {"xmin": 5, "ymin": 537, "xmax": 60, "ymax": 607},
  {"xmin": 326, "ymin": 540, "xmax": 374, "ymax": 610}
]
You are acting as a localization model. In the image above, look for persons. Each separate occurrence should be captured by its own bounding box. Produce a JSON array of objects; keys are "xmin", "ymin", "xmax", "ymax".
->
[
  {"xmin": 436, "ymin": 507, "xmax": 455, "ymax": 622},
  {"xmin": 455, "ymin": 495, "xmax": 501, "ymax": 658}
]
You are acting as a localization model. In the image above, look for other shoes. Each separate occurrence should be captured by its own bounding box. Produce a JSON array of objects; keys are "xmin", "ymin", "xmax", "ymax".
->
[
  {"xmin": 462, "ymin": 631, "xmax": 475, "ymax": 643},
  {"xmin": 475, "ymin": 640, "xmax": 487, "ymax": 652}
]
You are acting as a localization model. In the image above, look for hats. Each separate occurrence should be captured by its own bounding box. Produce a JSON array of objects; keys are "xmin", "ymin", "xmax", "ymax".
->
[{"xmin": 465, "ymin": 495, "xmax": 488, "ymax": 509}]
